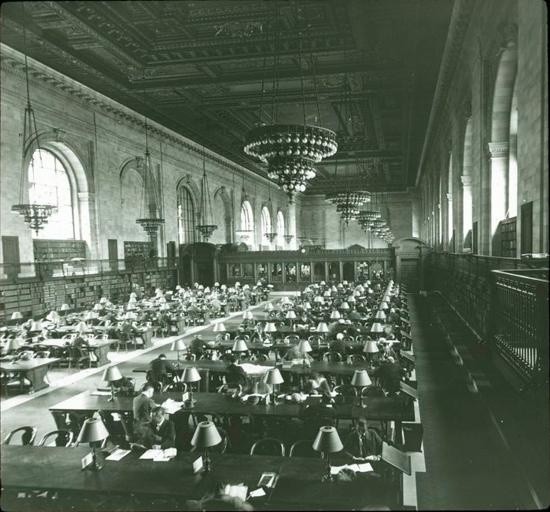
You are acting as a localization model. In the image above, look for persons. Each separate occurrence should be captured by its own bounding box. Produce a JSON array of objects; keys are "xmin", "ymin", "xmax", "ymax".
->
[{"xmin": 0, "ymin": 279, "xmax": 402, "ymax": 457}]
